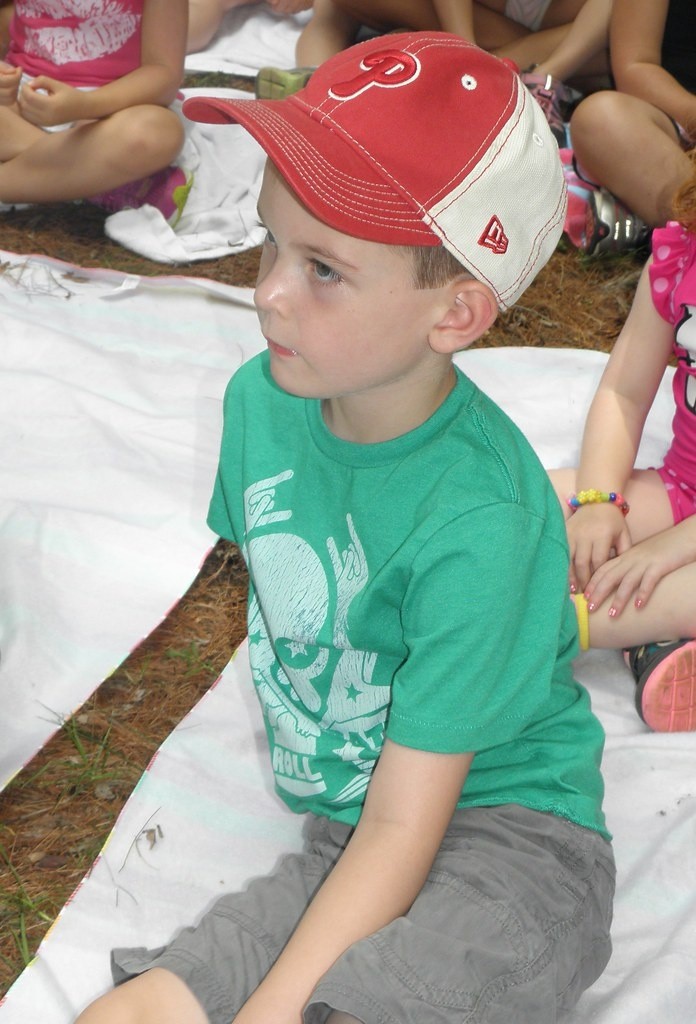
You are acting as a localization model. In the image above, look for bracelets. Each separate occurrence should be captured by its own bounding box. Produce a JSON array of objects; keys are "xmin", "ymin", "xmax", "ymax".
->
[{"xmin": 567, "ymin": 489, "xmax": 629, "ymax": 518}]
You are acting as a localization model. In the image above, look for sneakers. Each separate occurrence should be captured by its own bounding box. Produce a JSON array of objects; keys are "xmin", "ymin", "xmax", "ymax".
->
[
  {"xmin": 86, "ymin": 164, "xmax": 193, "ymax": 229},
  {"xmin": 519, "ymin": 64, "xmax": 568, "ymax": 150},
  {"xmin": 584, "ymin": 189, "xmax": 652, "ymax": 263}
]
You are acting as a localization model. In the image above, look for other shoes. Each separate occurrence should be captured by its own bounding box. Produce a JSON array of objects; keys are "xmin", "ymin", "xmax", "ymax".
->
[
  {"xmin": 255, "ymin": 67, "xmax": 314, "ymax": 100},
  {"xmin": 621, "ymin": 638, "xmax": 696, "ymax": 733}
]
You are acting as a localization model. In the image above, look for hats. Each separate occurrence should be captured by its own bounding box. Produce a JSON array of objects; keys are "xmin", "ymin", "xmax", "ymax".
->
[{"xmin": 181, "ymin": 30, "xmax": 568, "ymax": 313}]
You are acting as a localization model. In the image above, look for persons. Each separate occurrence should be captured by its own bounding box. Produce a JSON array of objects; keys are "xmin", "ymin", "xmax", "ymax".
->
[
  {"xmin": 545, "ymin": 148, "xmax": 696, "ymax": 734},
  {"xmin": 0, "ymin": 0, "xmax": 696, "ymax": 260},
  {"xmin": 73, "ymin": 32, "xmax": 615, "ymax": 1024}
]
